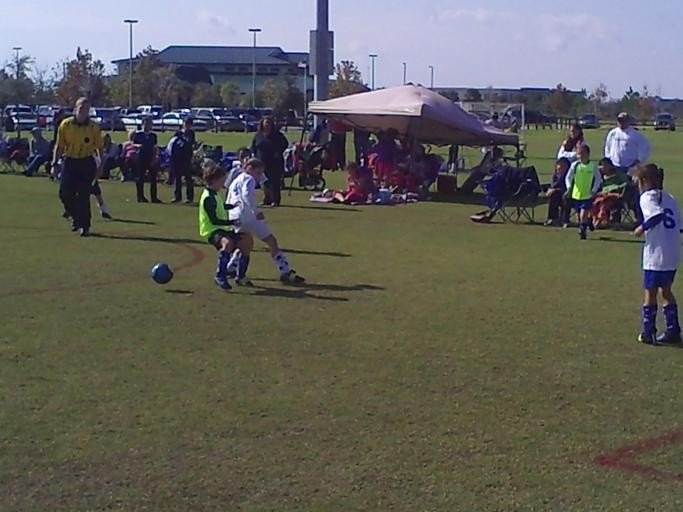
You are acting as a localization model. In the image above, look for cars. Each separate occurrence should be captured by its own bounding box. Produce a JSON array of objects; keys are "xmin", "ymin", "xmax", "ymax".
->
[
  {"xmin": 469, "ymin": 109, "xmax": 553, "ymax": 129},
  {"xmin": 0, "ymin": 106, "xmax": 276, "ymax": 134},
  {"xmin": 654, "ymin": 113, "xmax": 676, "ymax": 131},
  {"xmin": 577, "ymin": 113, "xmax": 599, "ymax": 129}
]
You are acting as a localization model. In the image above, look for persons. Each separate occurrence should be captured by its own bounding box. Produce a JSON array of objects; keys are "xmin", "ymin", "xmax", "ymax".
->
[
  {"xmin": 133, "ymin": 118, "xmax": 164, "ymax": 205},
  {"xmin": 457, "ymin": 140, "xmax": 504, "ymax": 194},
  {"xmin": 307, "ymin": 126, "xmax": 321, "ymax": 144},
  {"xmin": 169, "ymin": 117, "xmax": 195, "ymax": 203},
  {"xmin": 0, "ymin": 132, "xmax": 15, "ymax": 173},
  {"xmin": 192, "ymin": 139, "xmax": 307, "ymax": 292},
  {"xmin": 544, "ymin": 111, "xmax": 650, "ymax": 240},
  {"xmin": 53, "ymin": 131, "xmax": 140, "ymax": 183},
  {"xmin": 629, "ymin": 164, "xmax": 682, "ymax": 344},
  {"xmin": 324, "ymin": 128, "xmax": 428, "ymax": 204},
  {"xmin": 22, "ymin": 126, "xmax": 51, "ymax": 177},
  {"xmin": 50, "ymin": 96, "xmax": 113, "ymax": 239},
  {"xmin": 250, "ymin": 116, "xmax": 289, "ymax": 206}
]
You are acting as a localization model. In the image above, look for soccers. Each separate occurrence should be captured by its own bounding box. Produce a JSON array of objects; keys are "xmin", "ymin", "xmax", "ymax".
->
[{"xmin": 151, "ymin": 262, "xmax": 172, "ymax": 283}]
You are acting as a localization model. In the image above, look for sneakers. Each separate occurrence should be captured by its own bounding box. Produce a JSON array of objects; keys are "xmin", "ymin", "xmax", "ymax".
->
[
  {"xmin": 657, "ymin": 329, "xmax": 681, "ymax": 342},
  {"xmin": 280, "ymin": 269, "xmax": 305, "ymax": 282},
  {"xmin": 102, "ymin": 212, "xmax": 111, "ymax": 218},
  {"xmin": 214, "ymin": 270, "xmax": 253, "ymax": 289},
  {"xmin": 63, "ymin": 211, "xmax": 88, "ymax": 236},
  {"xmin": 587, "ymin": 216, "xmax": 594, "ymax": 231},
  {"xmin": 637, "ymin": 332, "xmax": 656, "ymax": 344},
  {"xmin": 544, "ymin": 219, "xmax": 570, "ymax": 229},
  {"xmin": 137, "ymin": 197, "xmax": 193, "ymax": 203},
  {"xmin": 580, "ymin": 230, "xmax": 586, "ymax": 239}
]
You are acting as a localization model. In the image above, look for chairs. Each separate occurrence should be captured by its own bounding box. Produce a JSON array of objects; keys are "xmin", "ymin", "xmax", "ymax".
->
[
  {"xmin": 500, "ymin": 165, "xmax": 635, "ymax": 229},
  {"xmin": 419, "ymin": 155, "xmax": 444, "ymax": 194}
]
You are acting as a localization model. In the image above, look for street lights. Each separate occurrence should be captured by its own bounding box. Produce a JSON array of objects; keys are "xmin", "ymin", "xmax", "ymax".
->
[
  {"xmin": 248, "ymin": 29, "xmax": 261, "ymax": 107},
  {"xmin": 13, "ymin": 47, "xmax": 21, "ymax": 77},
  {"xmin": 124, "ymin": 20, "xmax": 138, "ymax": 105},
  {"xmin": 369, "ymin": 54, "xmax": 377, "ymax": 90}
]
